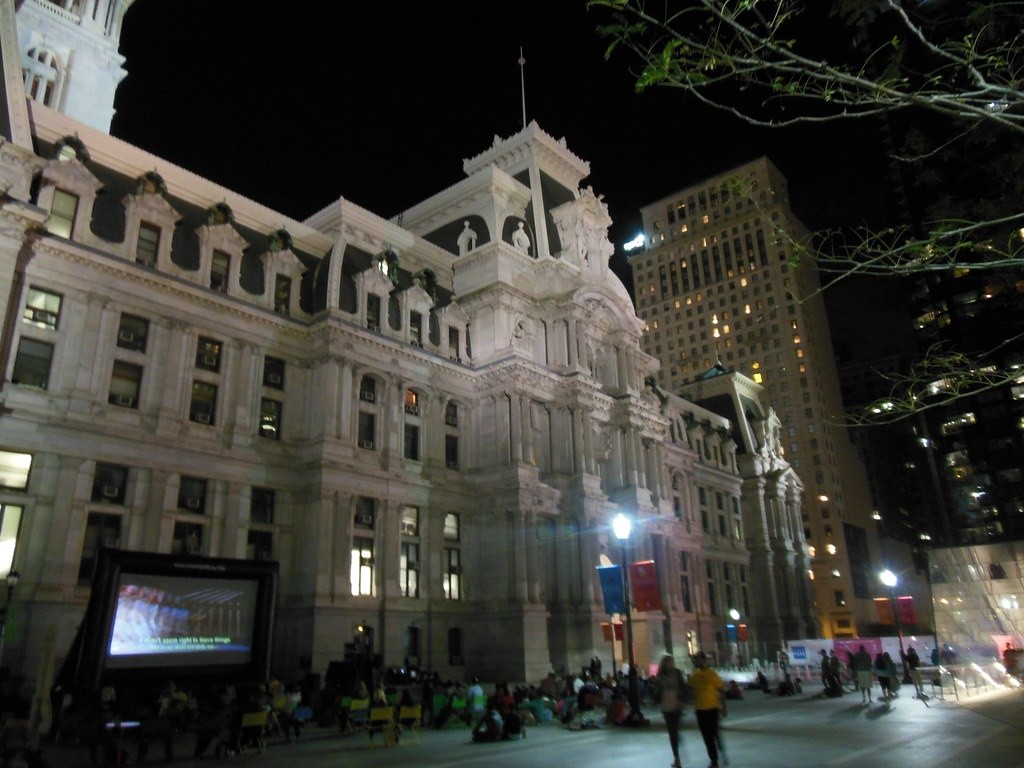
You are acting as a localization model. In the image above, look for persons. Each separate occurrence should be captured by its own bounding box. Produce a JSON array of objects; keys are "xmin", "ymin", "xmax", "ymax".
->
[
  {"xmin": 729, "ymin": 680, "xmax": 743, "ymax": 699},
  {"xmin": 845, "ymin": 648, "xmax": 858, "ymax": 692},
  {"xmin": 883, "ymin": 652, "xmax": 900, "ymax": 697},
  {"xmin": 908, "ymin": 645, "xmax": 913, "ymax": 652},
  {"xmin": 942, "ymin": 644, "xmax": 956, "ymax": 664},
  {"xmin": 138, "ymin": 679, "xmax": 187, "ymax": 761},
  {"xmin": 690, "ymin": 651, "xmax": 728, "ymax": 768},
  {"xmin": 830, "ymin": 649, "xmax": 842, "ymax": 685},
  {"xmin": 781, "ymin": 673, "xmax": 794, "ymax": 696},
  {"xmin": 901, "ymin": 649, "xmax": 925, "ymax": 696},
  {"xmin": 655, "ymin": 654, "xmax": 689, "ymax": 768},
  {"xmin": 81, "ymin": 686, "xmax": 123, "ymax": 765},
  {"xmin": 820, "ymin": 649, "xmax": 839, "ymax": 688},
  {"xmin": 931, "ymin": 648, "xmax": 939, "ymax": 665},
  {"xmin": 853, "ymin": 645, "xmax": 874, "ymax": 703},
  {"xmin": 194, "ymin": 653, "xmax": 656, "ymax": 759},
  {"xmin": 758, "ymin": 671, "xmax": 772, "ymax": 693},
  {"xmin": 794, "ymin": 677, "xmax": 801, "ymax": 693},
  {"xmin": 875, "ymin": 652, "xmax": 890, "ymax": 696}
]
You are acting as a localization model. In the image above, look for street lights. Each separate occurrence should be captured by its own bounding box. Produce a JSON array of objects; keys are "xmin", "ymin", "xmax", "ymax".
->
[
  {"xmin": 880, "ymin": 569, "xmax": 913, "ymax": 685},
  {"xmin": 612, "ymin": 511, "xmax": 651, "ymax": 730}
]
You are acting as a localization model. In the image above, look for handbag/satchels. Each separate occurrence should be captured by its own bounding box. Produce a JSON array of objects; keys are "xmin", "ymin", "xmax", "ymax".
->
[{"xmin": 650, "ymin": 687, "xmax": 662, "ymax": 705}]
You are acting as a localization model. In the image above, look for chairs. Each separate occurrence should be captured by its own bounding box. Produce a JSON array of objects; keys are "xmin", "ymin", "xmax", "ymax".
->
[
  {"xmin": 362, "ymin": 707, "xmax": 395, "ymax": 747},
  {"xmin": 448, "ymin": 697, "xmax": 467, "ymax": 729},
  {"xmin": 469, "ymin": 695, "xmax": 488, "ymax": 727},
  {"xmin": 396, "ymin": 705, "xmax": 422, "ymax": 747},
  {"xmin": 270, "ymin": 694, "xmax": 286, "ymax": 738},
  {"xmin": 340, "ymin": 697, "xmax": 370, "ymax": 738}
]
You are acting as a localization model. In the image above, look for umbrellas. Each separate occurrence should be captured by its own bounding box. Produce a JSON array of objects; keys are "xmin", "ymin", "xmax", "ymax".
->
[{"xmin": 27, "ymin": 625, "xmax": 56, "ymax": 751}]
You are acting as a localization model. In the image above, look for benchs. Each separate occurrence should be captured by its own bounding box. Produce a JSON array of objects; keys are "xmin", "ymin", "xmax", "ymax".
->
[{"xmin": 234, "ymin": 712, "xmax": 267, "ymax": 758}]
